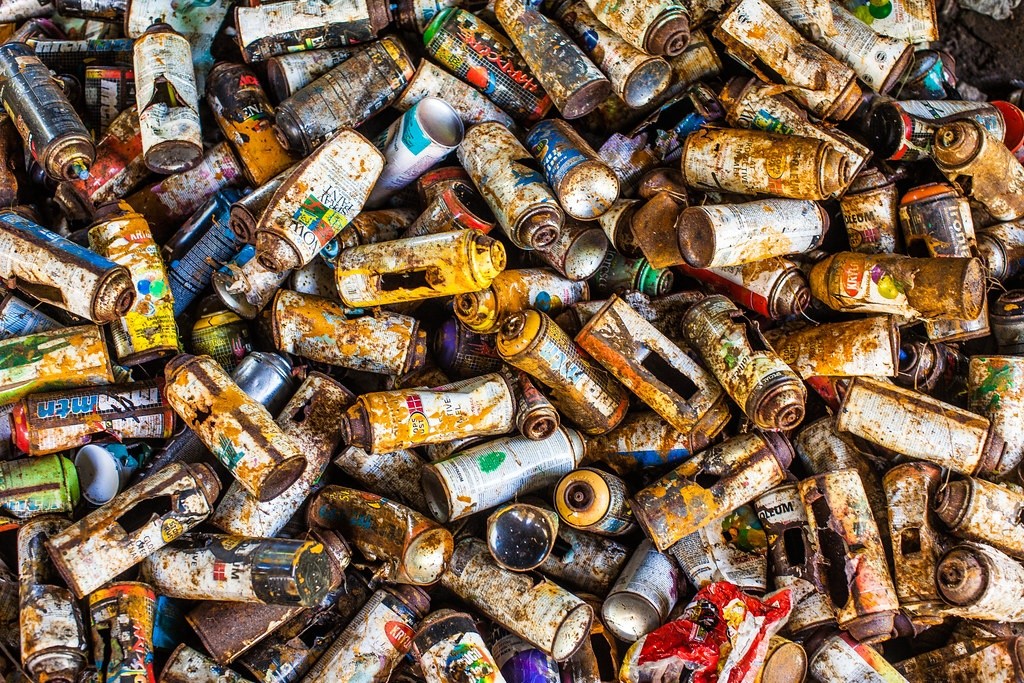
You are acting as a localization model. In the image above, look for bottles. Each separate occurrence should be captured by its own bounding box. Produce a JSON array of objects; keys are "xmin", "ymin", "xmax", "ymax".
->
[{"xmin": 1, "ymin": 1, "xmax": 1024, "ymax": 683}]
